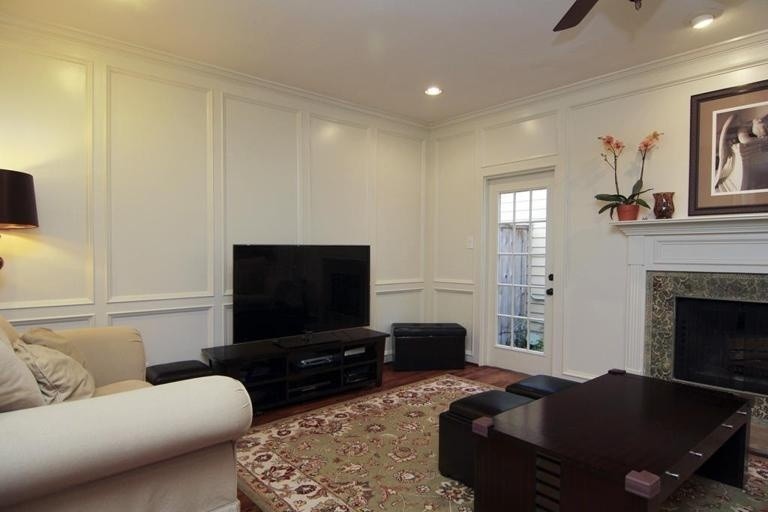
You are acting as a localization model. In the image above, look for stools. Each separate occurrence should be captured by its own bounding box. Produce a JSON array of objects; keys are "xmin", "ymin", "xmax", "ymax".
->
[
  {"xmin": 391, "ymin": 322, "xmax": 467, "ymax": 370},
  {"xmin": 436, "ymin": 374, "xmax": 581, "ymax": 489}
]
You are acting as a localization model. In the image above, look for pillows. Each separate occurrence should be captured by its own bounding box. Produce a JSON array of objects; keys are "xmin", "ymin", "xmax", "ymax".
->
[{"xmin": 13, "ymin": 326, "xmax": 96, "ymax": 402}]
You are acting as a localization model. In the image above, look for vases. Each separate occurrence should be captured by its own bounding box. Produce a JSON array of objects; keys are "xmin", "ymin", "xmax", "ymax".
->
[{"xmin": 616, "ymin": 191, "xmax": 676, "ymax": 221}]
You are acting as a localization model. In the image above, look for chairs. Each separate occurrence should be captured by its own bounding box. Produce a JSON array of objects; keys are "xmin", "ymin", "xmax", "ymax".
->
[{"xmin": 0, "ymin": 311, "xmax": 256, "ymax": 512}]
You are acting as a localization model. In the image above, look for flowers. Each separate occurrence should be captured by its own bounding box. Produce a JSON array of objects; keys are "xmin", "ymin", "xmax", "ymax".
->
[{"xmin": 591, "ymin": 130, "xmax": 667, "ymax": 221}]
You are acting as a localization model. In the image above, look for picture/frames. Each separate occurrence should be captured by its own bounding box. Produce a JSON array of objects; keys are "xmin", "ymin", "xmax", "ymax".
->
[{"xmin": 686, "ymin": 79, "xmax": 767, "ymax": 218}]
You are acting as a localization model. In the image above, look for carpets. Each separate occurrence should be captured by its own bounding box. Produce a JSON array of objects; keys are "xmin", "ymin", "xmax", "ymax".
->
[{"xmin": 234, "ymin": 371, "xmax": 767, "ymax": 511}]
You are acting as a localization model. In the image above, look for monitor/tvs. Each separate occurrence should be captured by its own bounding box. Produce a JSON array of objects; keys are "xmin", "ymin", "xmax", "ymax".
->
[{"xmin": 232, "ymin": 244, "xmax": 370, "ymax": 343}]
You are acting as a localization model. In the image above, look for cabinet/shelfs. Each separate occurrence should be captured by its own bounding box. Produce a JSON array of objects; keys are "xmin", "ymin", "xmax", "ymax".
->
[{"xmin": 201, "ymin": 326, "xmax": 391, "ymax": 415}]
